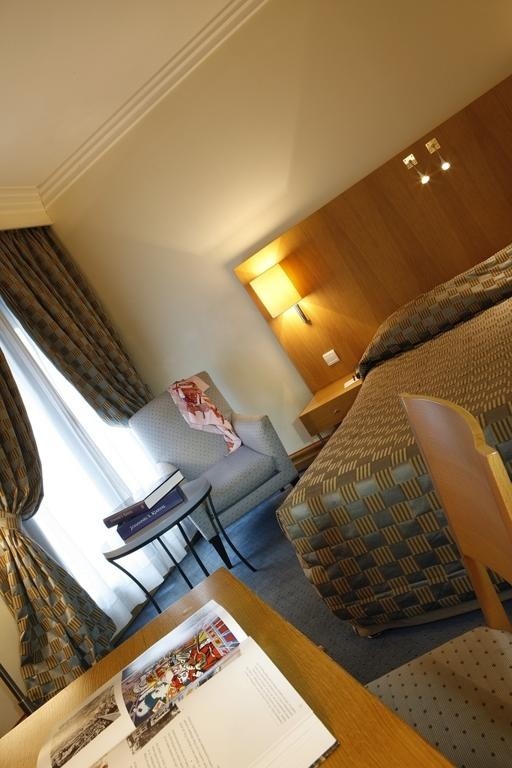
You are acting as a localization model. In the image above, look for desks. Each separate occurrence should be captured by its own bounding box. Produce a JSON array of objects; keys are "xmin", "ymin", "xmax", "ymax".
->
[
  {"xmin": 2, "ymin": 565, "xmax": 473, "ymax": 766},
  {"xmin": 99, "ymin": 475, "xmax": 261, "ymax": 615}
]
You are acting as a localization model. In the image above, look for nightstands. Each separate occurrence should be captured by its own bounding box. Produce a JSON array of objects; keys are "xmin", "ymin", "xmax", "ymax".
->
[{"xmin": 296, "ymin": 368, "xmax": 369, "ymax": 451}]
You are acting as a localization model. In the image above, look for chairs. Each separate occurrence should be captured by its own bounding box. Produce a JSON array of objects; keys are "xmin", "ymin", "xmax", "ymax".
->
[
  {"xmin": 367, "ymin": 385, "xmax": 512, "ymax": 766},
  {"xmin": 125, "ymin": 369, "xmax": 305, "ymax": 573}
]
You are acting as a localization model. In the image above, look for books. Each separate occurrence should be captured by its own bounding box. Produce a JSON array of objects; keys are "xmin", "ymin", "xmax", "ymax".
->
[
  {"xmin": 104, "ymin": 467, "xmax": 186, "ymax": 528},
  {"xmin": 117, "ymin": 485, "xmax": 186, "ymax": 542},
  {"xmin": 35, "ymin": 598, "xmax": 341, "ymax": 768}
]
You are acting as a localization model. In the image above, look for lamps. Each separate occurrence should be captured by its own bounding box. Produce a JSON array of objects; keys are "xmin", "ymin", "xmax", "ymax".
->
[
  {"xmin": 246, "ymin": 261, "xmax": 315, "ymax": 328},
  {"xmin": 424, "ymin": 137, "xmax": 453, "ymax": 174},
  {"xmin": 401, "ymin": 152, "xmax": 430, "ymax": 183}
]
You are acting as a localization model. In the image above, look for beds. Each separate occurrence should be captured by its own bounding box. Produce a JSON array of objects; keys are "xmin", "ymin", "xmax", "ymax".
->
[{"xmin": 272, "ymin": 239, "xmax": 512, "ymax": 639}]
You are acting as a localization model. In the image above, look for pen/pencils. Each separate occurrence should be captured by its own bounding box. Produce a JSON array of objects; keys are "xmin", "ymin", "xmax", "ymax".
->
[{"xmin": 352, "ymin": 374, "xmax": 356, "ymax": 381}]
